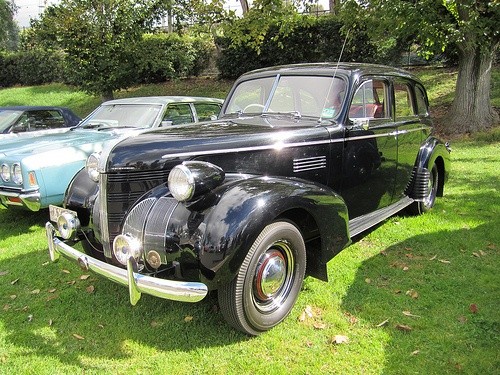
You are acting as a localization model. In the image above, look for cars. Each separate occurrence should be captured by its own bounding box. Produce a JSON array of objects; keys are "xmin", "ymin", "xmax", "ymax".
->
[
  {"xmin": 0, "ymin": 106, "xmax": 82, "ymax": 134},
  {"xmin": 0, "ymin": 96, "xmax": 225, "ymax": 211},
  {"xmin": 44, "ymin": 60, "xmax": 451, "ymax": 335}
]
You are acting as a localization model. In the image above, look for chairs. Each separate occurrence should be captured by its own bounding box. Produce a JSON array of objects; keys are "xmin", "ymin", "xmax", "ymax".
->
[{"xmin": 332, "ymin": 103, "xmax": 383, "ymax": 118}]
